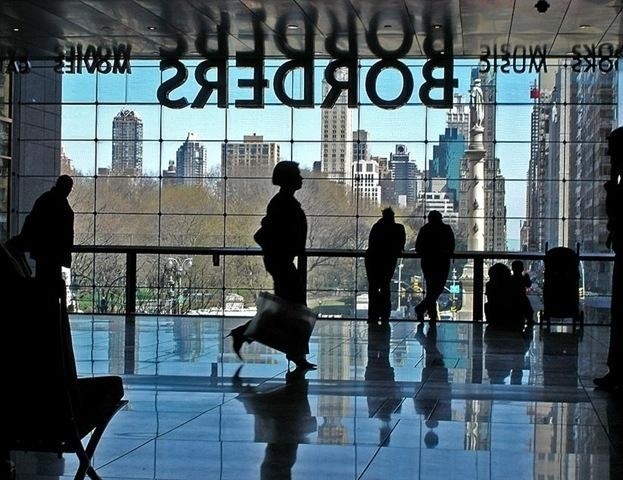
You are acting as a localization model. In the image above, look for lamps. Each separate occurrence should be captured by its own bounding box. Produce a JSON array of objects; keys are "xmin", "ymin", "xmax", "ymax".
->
[{"xmin": 0, "ymin": 22, "xmax": 32, "ymax": 75}]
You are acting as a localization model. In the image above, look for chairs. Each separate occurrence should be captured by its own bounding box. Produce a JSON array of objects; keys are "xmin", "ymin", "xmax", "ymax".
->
[{"xmin": 0, "ymin": 279, "xmax": 128, "ymax": 480}]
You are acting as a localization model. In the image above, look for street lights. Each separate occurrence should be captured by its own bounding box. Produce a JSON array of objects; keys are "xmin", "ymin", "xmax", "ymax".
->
[
  {"xmin": 406, "ymin": 293, "xmax": 413, "ymax": 320},
  {"xmin": 164, "ymin": 256, "xmax": 194, "ymax": 314},
  {"xmin": 317, "ymin": 299, "xmax": 323, "ymax": 314},
  {"xmin": 66, "ymin": 267, "xmax": 126, "ymax": 314},
  {"xmin": 396, "ymin": 247, "xmax": 416, "ymax": 312}
]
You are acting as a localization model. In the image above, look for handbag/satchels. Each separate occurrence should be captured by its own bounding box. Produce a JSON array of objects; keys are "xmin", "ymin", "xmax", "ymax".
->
[{"xmin": 243, "ymin": 291, "xmax": 318, "ymax": 355}]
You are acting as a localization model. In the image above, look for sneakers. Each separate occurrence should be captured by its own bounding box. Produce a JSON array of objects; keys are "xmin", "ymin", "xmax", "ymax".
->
[{"xmin": 414, "ymin": 303, "xmax": 425, "ymax": 324}]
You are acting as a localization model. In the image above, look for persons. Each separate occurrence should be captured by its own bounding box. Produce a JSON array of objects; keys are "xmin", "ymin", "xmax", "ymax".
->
[
  {"xmin": 364, "ymin": 322, "xmax": 395, "ymax": 424},
  {"xmin": 481, "ymin": 325, "xmax": 534, "ymax": 386},
  {"xmin": 483, "ymin": 263, "xmax": 527, "ymax": 333},
  {"xmin": 412, "ymin": 208, "xmax": 457, "ymax": 322},
  {"xmin": 511, "ymin": 260, "xmax": 535, "ymax": 325},
  {"xmin": 363, "ymin": 205, "xmax": 406, "ymax": 326},
  {"xmin": 220, "ymin": 159, "xmax": 319, "ymax": 369},
  {"xmin": 592, "ymin": 125, "xmax": 623, "ymax": 390},
  {"xmin": 18, "ymin": 173, "xmax": 75, "ymax": 304},
  {"xmin": 413, "ymin": 321, "xmax": 446, "ymax": 429},
  {"xmin": 229, "ymin": 364, "xmax": 311, "ymax": 480}
]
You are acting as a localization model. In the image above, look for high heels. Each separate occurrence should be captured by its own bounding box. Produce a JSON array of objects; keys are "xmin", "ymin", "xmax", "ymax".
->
[
  {"xmin": 286, "ymin": 354, "xmax": 317, "ymax": 367},
  {"xmin": 226, "ymin": 326, "xmax": 245, "ymax": 360}
]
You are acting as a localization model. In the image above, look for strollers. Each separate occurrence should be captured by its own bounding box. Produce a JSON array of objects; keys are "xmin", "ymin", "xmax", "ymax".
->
[{"xmin": 538, "ymin": 240, "xmax": 587, "ymax": 331}]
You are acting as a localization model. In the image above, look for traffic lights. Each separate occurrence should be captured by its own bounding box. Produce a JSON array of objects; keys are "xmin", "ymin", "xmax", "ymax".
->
[
  {"xmin": 411, "ymin": 276, "xmax": 419, "ymax": 293},
  {"xmin": 400, "ymin": 287, "xmax": 407, "ymax": 299}
]
